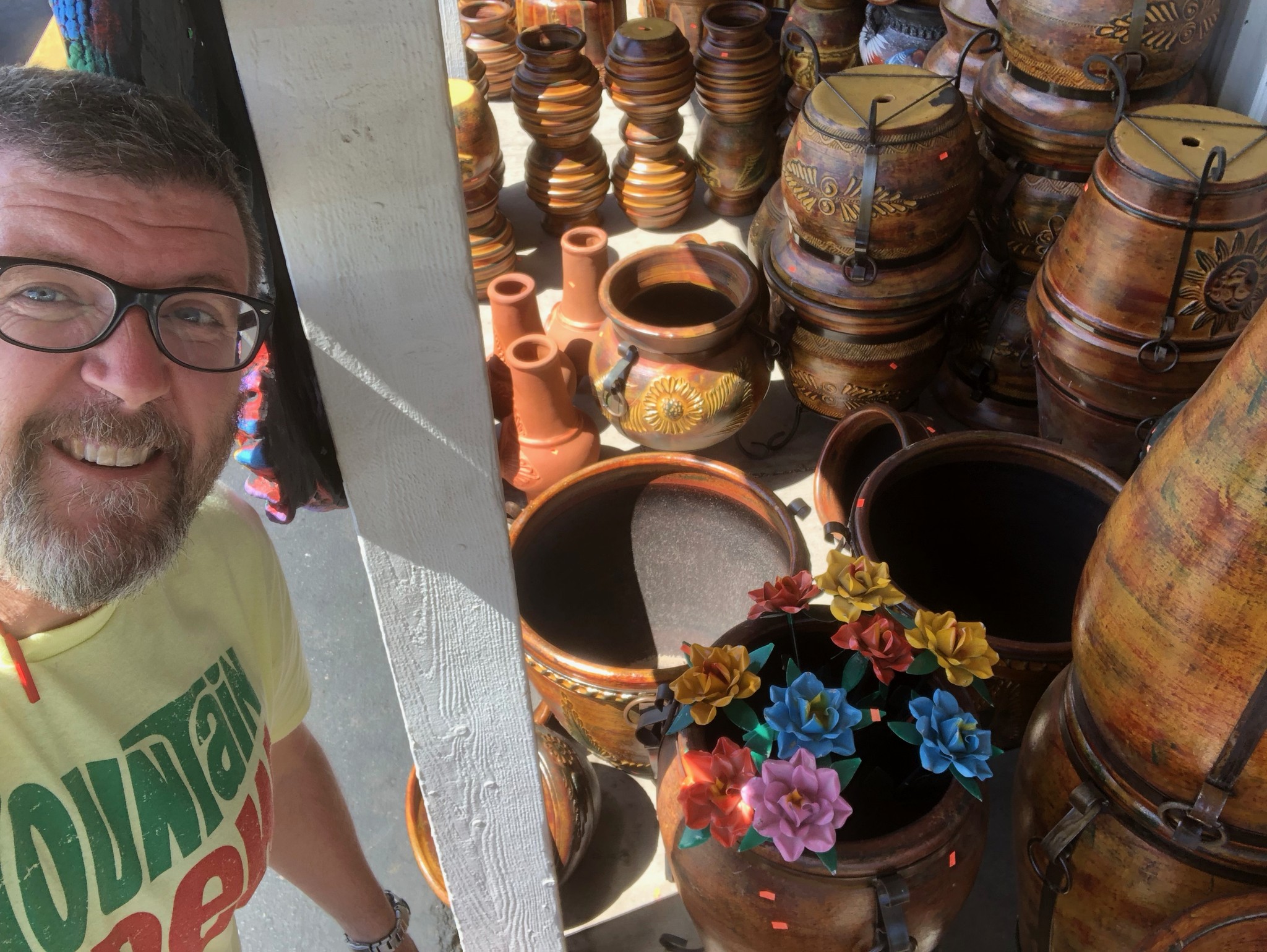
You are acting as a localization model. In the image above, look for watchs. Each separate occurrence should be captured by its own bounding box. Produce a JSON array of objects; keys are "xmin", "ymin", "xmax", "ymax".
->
[{"xmin": 344, "ymin": 890, "xmax": 411, "ymax": 952}]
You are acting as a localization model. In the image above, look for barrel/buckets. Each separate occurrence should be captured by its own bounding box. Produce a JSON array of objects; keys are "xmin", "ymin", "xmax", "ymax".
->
[{"xmin": 405, "ymin": 0, "xmax": 1267, "ymax": 952}]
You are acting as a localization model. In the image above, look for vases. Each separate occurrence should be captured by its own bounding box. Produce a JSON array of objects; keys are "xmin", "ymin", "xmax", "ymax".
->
[{"xmin": 407, "ymin": 0, "xmax": 1267, "ymax": 952}]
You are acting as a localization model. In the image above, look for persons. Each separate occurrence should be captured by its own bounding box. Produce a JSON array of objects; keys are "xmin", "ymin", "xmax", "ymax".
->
[{"xmin": 0, "ymin": 65, "xmax": 422, "ymax": 952}]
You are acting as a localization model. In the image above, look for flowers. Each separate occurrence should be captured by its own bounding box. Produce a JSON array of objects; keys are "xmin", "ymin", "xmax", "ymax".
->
[
  {"xmin": 742, "ymin": 656, "xmax": 885, "ymax": 759},
  {"xmin": 747, "ymin": 570, "xmax": 823, "ymax": 670},
  {"xmin": 802, "ymin": 550, "xmax": 906, "ymax": 624},
  {"xmin": 740, "ymin": 748, "xmax": 862, "ymax": 878},
  {"xmin": 829, "ymin": 608, "xmax": 923, "ymax": 692},
  {"xmin": 677, "ymin": 728, "xmax": 776, "ymax": 850},
  {"xmin": 666, "ymin": 642, "xmax": 775, "ymax": 736},
  {"xmin": 887, "ymin": 688, "xmax": 1004, "ymax": 802},
  {"xmin": 883, "ymin": 605, "xmax": 1000, "ymax": 708}
]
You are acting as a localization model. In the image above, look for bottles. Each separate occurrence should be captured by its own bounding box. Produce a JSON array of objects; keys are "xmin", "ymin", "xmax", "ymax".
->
[
  {"xmin": 484, "ymin": 272, "xmax": 577, "ymax": 423},
  {"xmin": 497, "ymin": 334, "xmax": 601, "ymax": 506},
  {"xmin": 544, "ymin": 225, "xmax": 608, "ymax": 395}
]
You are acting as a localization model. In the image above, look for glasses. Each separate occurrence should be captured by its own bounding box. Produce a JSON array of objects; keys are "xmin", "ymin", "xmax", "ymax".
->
[{"xmin": 0, "ymin": 256, "xmax": 276, "ymax": 373}]
